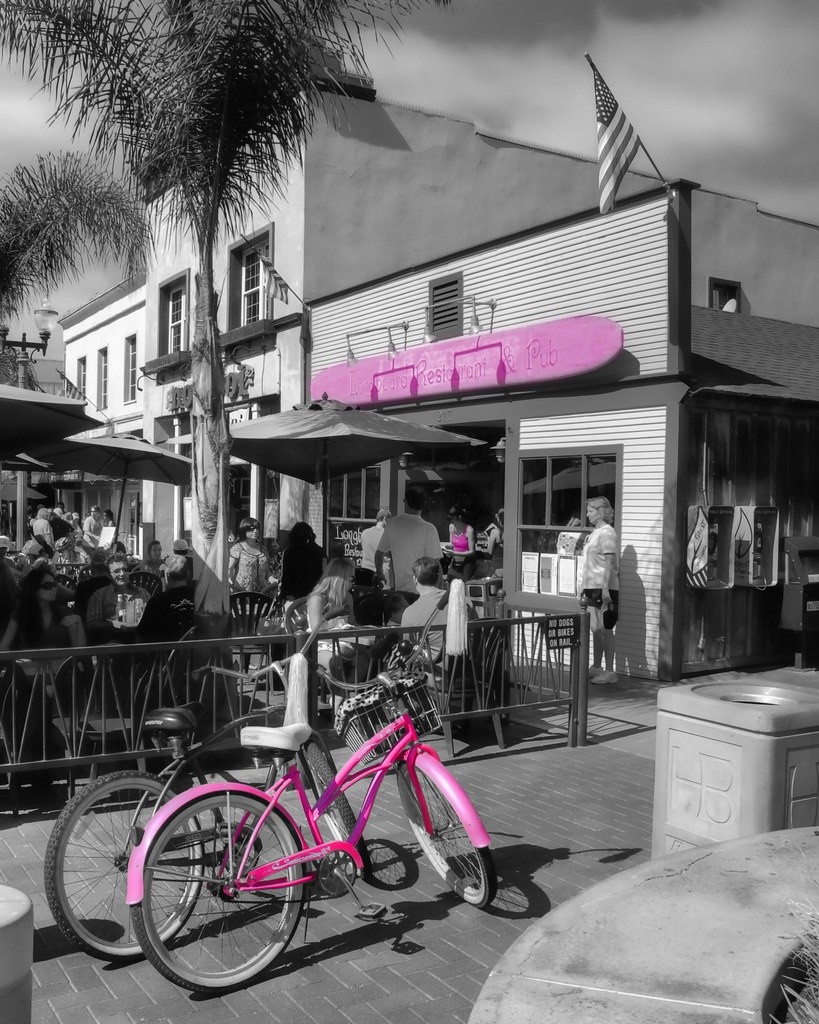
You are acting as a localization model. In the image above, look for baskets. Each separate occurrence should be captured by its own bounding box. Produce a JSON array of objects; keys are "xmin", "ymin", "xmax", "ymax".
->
[{"xmin": 333, "ymin": 675, "xmax": 442, "ymax": 767}]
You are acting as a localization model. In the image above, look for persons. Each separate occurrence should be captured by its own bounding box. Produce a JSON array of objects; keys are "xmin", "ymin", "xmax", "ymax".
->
[
  {"xmin": 0, "ymin": 502, "xmax": 193, "ymax": 651},
  {"xmin": 360, "ymin": 509, "xmax": 392, "ymax": 572},
  {"xmin": 228, "ymin": 518, "xmax": 327, "ymax": 603},
  {"xmin": 486, "ymin": 508, "xmax": 504, "ymax": 577},
  {"xmin": 374, "ymin": 486, "xmax": 444, "ymax": 594},
  {"xmin": 443, "ymin": 506, "xmax": 475, "ymax": 583},
  {"xmin": 306, "ymin": 557, "xmax": 370, "ymax": 715},
  {"xmin": 401, "ymin": 556, "xmax": 482, "ymax": 692},
  {"xmin": 579, "ymin": 497, "xmax": 620, "ymax": 686}
]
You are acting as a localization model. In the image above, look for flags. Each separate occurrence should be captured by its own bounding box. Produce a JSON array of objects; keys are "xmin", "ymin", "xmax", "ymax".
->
[
  {"xmin": 593, "ymin": 68, "xmax": 641, "ymax": 215},
  {"xmin": 260, "ymin": 255, "xmax": 289, "ymax": 305}
]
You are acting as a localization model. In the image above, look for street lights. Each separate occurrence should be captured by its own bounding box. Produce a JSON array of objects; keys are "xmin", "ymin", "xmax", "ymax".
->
[{"xmin": 0, "ymin": 298, "xmax": 58, "ymax": 551}]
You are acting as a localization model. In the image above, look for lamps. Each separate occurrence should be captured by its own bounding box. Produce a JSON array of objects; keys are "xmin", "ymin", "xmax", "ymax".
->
[
  {"xmin": 345, "ymin": 321, "xmax": 409, "ymax": 367},
  {"xmin": 136, "ymin": 344, "xmax": 252, "ymax": 391},
  {"xmin": 423, "ymin": 295, "xmax": 497, "ymax": 345}
]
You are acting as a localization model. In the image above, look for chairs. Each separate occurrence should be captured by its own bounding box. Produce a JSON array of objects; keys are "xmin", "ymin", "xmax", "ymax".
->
[{"xmin": 0, "ymin": 551, "xmax": 508, "ymax": 809}]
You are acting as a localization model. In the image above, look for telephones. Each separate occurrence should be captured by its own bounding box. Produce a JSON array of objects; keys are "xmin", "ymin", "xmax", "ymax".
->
[
  {"xmin": 753, "ymin": 522, "xmax": 764, "ymax": 561},
  {"xmin": 708, "ymin": 522, "xmax": 720, "ymax": 560}
]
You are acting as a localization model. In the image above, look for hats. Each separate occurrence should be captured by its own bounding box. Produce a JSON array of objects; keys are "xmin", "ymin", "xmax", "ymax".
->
[
  {"xmin": 71, "ymin": 513, "xmax": 79, "ymax": 520},
  {"xmin": 447, "ymin": 506, "xmax": 464, "ymax": 520},
  {"xmin": 159, "ymin": 555, "xmax": 188, "ymax": 575},
  {"xmin": 0, "ymin": 535, "xmax": 9, "ymax": 548},
  {"xmin": 172, "ymin": 539, "xmax": 189, "ymax": 551}
]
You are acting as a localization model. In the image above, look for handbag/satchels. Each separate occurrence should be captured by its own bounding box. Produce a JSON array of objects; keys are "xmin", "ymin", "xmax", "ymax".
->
[{"xmin": 603, "ymin": 603, "xmax": 617, "ymax": 630}]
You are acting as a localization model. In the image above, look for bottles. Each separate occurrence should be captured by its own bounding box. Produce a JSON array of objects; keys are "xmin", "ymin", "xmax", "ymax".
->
[
  {"xmin": 136, "ymin": 602, "xmax": 142, "ymax": 622},
  {"xmin": 116, "ymin": 594, "xmax": 125, "ymax": 623}
]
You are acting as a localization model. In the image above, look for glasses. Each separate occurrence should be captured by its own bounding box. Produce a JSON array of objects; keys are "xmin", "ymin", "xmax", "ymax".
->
[
  {"xmin": 347, "ymin": 576, "xmax": 354, "ymax": 583},
  {"xmin": 250, "ymin": 525, "xmax": 257, "ymax": 530},
  {"xmin": 38, "ymin": 582, "xmax": 56, "ymax": 590}
]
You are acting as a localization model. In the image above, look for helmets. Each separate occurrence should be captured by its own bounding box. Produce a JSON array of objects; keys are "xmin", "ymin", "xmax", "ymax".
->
[{"xmin": 54, "ymin": 537, "xmax": 73, "ymax": 550}]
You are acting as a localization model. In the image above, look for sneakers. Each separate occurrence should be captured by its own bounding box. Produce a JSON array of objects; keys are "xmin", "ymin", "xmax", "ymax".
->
[
  {"xmin": 588, "ymin": 666, "xmax": 604, "ymax": 677},
  {"xmin": 591, "ymin": 670, "xmax": 618, "ymax": 684}
]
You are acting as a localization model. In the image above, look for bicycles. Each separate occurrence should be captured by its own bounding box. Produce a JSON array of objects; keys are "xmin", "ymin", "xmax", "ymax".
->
[
  {"xmin": 127, "ymin": 587, "xmax": 498, "ymax": 996},
  {"xmin": 44, "ymin": 604, "xmax": 371, "ymax": 963}
]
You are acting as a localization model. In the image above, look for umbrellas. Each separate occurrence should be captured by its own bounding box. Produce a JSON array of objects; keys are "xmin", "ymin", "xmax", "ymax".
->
[
  {"xmin": 0, "ymin": 384, "xmax": 105, "ymax": 461},
  {"xmin": 228, "ymin": 393, "xmax": 487, "ymax": 552},
  {"xmin": 26, "ymin": 432, "xmax": 193, "ymax": 544}
]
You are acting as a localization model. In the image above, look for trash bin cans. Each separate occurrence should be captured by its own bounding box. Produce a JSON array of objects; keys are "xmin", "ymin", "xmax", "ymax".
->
[{"xmin": 648, "ymin": 667, "xmax": 819, "ymax": 864}]
